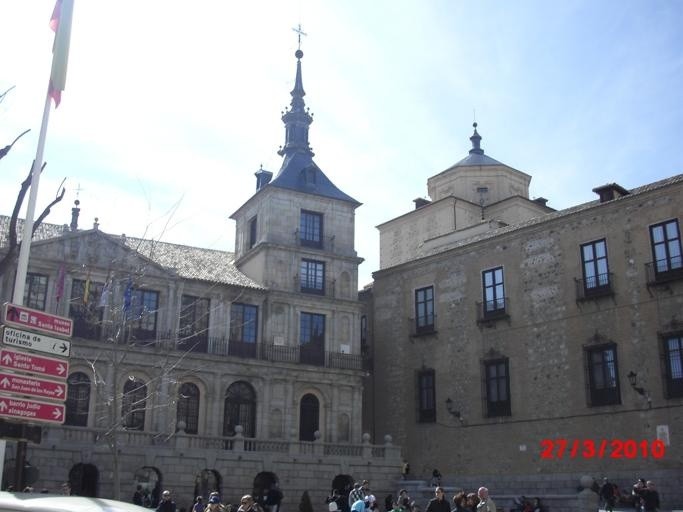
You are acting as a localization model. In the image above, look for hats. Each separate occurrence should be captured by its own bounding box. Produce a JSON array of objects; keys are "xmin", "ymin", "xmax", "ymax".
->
[{"xmin": 209, "ymin": 496, "xmax": 220, "ymax": 503}]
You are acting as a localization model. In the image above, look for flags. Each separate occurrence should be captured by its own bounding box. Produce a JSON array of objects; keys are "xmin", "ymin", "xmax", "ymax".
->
[
  {"xmin": 119, "ymin": 274, "xmax": 134, "ymax": 312},
  {"xmin": 44, "ymin": 0, "xmax": 76, "ymax": 108},
  {"xmin": 52, "ymin": 260, "xmax": 66, "ymax": 306},
  {"xmin": 83, "ymin": 273, "xmax": 90, "ymax": 306},
  {"xmin": 96, "ymin": 277, "xmax": 115, "ymax": 310}
]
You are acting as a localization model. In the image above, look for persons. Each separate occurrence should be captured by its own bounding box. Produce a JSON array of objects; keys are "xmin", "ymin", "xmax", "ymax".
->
[
  {"xmin": 592, "ymin": 477, "xmax": 662, "ymax": 512},
  {"xmin": 132, "ymin": 481, "xmax": 283, "ymax": 512},
  {"xmin": 326, "ymin": 479, "xmax": 496, "ymax": 512},
  {"xmin": 299, "ymin": 491, "xmax": 314, "ymax": 512},
  {"xmin": 7, "ymin": 483, "xmax": 17, "ymax": 493},
  {"xmin": 60, "ymin": 482, "xmax": 72, "ymax": 496},
  {"xmin": 510, "ymin": 497, "xmax": 545, "ymax": 512}
]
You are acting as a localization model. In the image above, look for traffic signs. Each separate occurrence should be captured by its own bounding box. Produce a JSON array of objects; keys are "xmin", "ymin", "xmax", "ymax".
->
[
  {"xmin": 0, "ymin": 395, "xmax": 66, "ymax": 425},
  {"xmin": 0, "ymin": 347, "xmax": 68, "ymax": 380},
  {"xmin": 0, "ymin": 324, "xmax": 70, "ymax": 358},
  {"xmin": 2, "ymin": 301, "xmax": 72, "ymax": 338},
  {"xmin": 0, "ymin": 371, "xmax": 68, "ymax": 401}
]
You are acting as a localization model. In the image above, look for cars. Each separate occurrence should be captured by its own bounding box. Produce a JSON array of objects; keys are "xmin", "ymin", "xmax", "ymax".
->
[{"xmin": 0, "ymin": 490, "xmax": 157, "ymax": 512}]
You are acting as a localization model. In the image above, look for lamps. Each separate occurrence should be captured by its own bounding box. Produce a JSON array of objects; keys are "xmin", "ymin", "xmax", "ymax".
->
[
  {"xmin": 444, "ymin": 397, "xmax": 464, "ymax": 426},
  {"xmin": 625, "ymin": 370, "xmax": 652, "ymax": 410}
]
